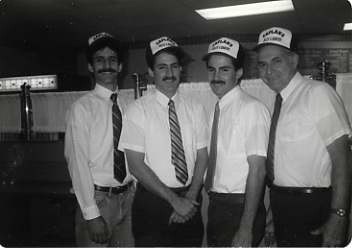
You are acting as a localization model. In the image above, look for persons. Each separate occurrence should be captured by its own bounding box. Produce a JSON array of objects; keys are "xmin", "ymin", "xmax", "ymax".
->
[
  {"xmin": 64, "ymin": 32, "xmax": 139, "ymax": 248},
  {"xmin": 252, "ymin": 27, "xmax": 352, "ymax": 248},
  {"xmin": 202, "ymin": 37, "xmax": 273, "ymax": 248},
  {"xmin": 117, "ymin": 37, "xmax": 209, "ymax": 247}
]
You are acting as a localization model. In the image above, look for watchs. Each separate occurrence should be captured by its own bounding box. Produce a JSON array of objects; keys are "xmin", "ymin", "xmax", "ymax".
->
[{"xmin": 329, "ymin": 208, "xmax": 349, "ymax": 217}]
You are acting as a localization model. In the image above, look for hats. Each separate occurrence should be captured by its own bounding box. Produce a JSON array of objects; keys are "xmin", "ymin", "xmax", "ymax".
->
[
  {"xmin": 146, "ymin": 35, "xmax": 181, "ymax": 63},
  {"xmin": 202, "ymin": 36, "xmax": 244, "ymax": 64},
  {"xmin": 88, "ymin": 31, "xmax": 121, "ymax": 53},
  {"xmin": 253, "ymin": 26, "xmax": 297, "ymax": 54}
]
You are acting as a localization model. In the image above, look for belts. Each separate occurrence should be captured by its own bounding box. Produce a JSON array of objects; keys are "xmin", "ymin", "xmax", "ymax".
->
[
  {"xmin": 209, "ymin": 191, "xmax": 245, "ymax": 202},
  {"xmin": 271, "ymin": 184, "xmax": 332, "ymax": 194},
  {"xmin": 94, "ymin": 180, "xmax": 134, "ymax": 194}
]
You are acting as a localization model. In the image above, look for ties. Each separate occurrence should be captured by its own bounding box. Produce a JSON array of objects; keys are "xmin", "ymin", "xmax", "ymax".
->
[
  {"xmin": 110, "ymin": 93, "xmax": 127, "ymax": 183},
  {"xmin": 168, "ymin": 99, "xmax": 188, "ymax": 186},
  {"xmin": 203, "ymin": 101, "xmax": 220, "ymax": 191},
  {"xmin": 266, "ymin": 93, "xmax": 282, "ymax": 188}
]
